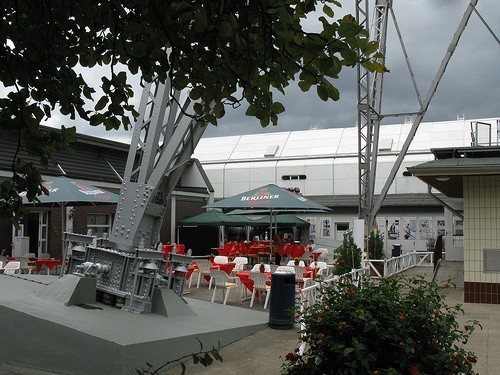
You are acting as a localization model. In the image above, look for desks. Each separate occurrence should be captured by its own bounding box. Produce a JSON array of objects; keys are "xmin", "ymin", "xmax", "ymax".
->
[
  {"xmin": 303, "ymin": 266, "xmax": 321, "ymax": 281},
  {"xmin": 36, "ymin": 259, "xmax": 62, "ymax": 275},
  {"xmin": 191, "ymin": 263, "xmax": 236, "ymax": 288},
  {"xmin": 310, "ymin": 251, "xmax": 323, "ymax": 261},
  {"xmin": 235, "ymin": 271, "xmax": 272, "ymax": 305},
  {"xmin": 7, "ymin": 256, "xmax": 36, "ymax": 265}
]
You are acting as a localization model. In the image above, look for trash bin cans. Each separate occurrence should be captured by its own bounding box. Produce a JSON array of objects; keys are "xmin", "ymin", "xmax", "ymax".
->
[
  {"xmin": 392, "ymin": 244, "xmax": 402, "ymax": 257},
  {"xmin": 269, "ymin": 272, "xmax": 296, "ymax": 330}
]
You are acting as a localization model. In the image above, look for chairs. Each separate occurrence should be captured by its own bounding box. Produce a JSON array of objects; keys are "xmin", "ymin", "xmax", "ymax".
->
[
  {"xmin": 160, "ymin": 240, "xmax": 333, "ymax": 311},
  {"xmin": 0, "ymin": 252, "xmax": 51, "ymax": 276}
]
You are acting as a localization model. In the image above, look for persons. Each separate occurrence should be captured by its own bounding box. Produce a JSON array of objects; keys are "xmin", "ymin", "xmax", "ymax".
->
[
  {"xmin": 287, "ymin": 233, "xmax": 294, "ymax": 244},
  {"xmin": 295, "ymin": 229, "xmax": 302, "ymax": 241},
  {"xmin": 390, "ymin": 222, "xmax": 395, "ymax": 233},
  {"xmin": 305, "ymin": 240, "xmax": 315, "ymax": 251},
  {"xmin": 274, "ymin": 229, "xmax": 284, "ymax": 266}
]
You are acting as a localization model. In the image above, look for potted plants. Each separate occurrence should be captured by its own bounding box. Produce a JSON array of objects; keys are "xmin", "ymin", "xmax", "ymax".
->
[
  {"xmin": 258, "ymin": 261, "xmax": 266, "ymax": 273},
  {"xmin": 293, "ymin": 256, "xmax": 302, "ymax": 267},
  {"xmin": 426, "ymin": 237, "xmax": 436, "ymax": 252}
]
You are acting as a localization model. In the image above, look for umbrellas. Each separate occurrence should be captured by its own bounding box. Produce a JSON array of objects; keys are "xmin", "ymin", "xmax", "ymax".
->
[
  {"xmin": 201, "ymin": 184, "xmax": 333, "ymax": 263},
  {"xmin": 256, "ymin": 214, "xmax": 310, "ymax": 227},
  {"xmin": 0, "ymin": 176, "xmax": 119, "ymax": 257},
  {"xmin": 178, "ymin": 209, "xmax": 252, "ymax": 249}
]
